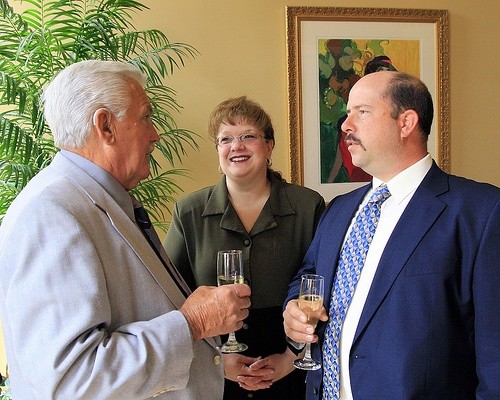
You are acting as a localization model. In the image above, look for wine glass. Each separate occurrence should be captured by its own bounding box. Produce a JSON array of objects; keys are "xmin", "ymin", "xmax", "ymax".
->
[
  {"xmin": 216, "ymin": 250, "xmax": 248, "ymax": 353},
  {"xmin": 292, "ymin": 274, "xmax": 326, "ymax": 370}
]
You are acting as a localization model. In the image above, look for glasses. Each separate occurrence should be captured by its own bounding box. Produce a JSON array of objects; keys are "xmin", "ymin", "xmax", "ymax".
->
[{"xmin": 214, "ymin": 132, "xmax": 267, "ymax": 150}]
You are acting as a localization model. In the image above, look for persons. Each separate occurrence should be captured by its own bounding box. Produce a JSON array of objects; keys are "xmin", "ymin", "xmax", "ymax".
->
[
  {"xmin": 0, "ymin": 60, "xmax": 252, "ymax": 400},
  {"xmin": 283, "ymin": 71, "xmax": 500, "ymax": 400},
  {"xmin": 164, "ymin": 96, "xmax": 326, "ymax": 400}
]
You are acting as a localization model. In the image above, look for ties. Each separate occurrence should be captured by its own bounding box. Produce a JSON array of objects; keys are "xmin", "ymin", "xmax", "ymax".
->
[
  {"xmin": 131, "ymin": 194, "xmax": 191, "ymax": 296},
  {"xmin": 322, "ymin": 183, "xmax": 391, "ymax": 400}
]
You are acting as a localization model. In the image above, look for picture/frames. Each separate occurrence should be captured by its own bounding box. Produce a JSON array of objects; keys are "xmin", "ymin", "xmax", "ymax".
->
[{"xmin": 284, "ymin": 5, "xmax": 452, "ymax": 207}]
